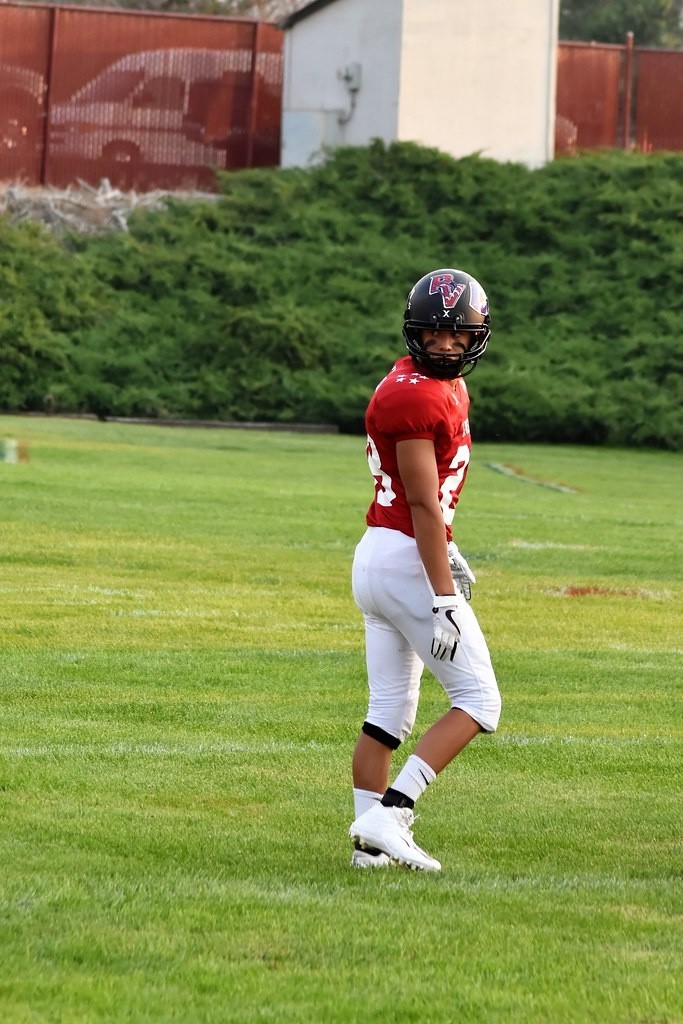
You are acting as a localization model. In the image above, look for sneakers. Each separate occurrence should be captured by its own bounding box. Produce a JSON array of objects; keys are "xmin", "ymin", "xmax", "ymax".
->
[{"xmin": 348, "ymin": 806, "xmax": 441, "ymax": 873}]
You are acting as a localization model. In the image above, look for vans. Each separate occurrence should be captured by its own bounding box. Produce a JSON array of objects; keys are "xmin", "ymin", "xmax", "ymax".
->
[{"xmin": 37, "ymin": 48, "xmax": 284, "ymax": 174}]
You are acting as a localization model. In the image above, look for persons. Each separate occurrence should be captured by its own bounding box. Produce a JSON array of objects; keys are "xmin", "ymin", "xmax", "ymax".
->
[{"xmin": 349, "ymin": 268, "xmax": 502, "ymax": 871}]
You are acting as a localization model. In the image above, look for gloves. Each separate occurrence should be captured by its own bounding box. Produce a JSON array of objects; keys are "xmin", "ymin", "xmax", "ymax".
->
[
  {"xmin": 431, "ymin": 594, "xmax": 462, "ymax": 662},
  {"xmin": 447, "ymin": 540, "xmax": 476, "ymax": 601}
]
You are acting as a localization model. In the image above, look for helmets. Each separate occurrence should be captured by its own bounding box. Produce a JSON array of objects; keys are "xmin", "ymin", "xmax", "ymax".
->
[{"xmin": 402, "ymin": 268, "xmax": 492, "ymax": 381}]
